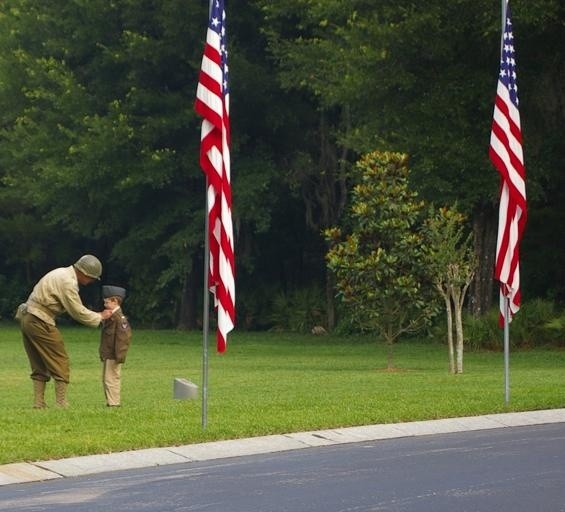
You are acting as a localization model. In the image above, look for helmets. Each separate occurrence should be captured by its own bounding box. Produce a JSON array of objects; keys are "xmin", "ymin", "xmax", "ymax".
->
[{"xmin": 73, "ymin": 254, "xmax": 103, "ymax": 280}]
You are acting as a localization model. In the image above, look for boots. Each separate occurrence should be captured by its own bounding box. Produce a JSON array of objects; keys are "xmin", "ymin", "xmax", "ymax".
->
[
  {"xmin": 55, "ymin": 382, "xmax": 70, "ymax": 408},
  {"xmin": 33, "ymin": 379, "xmax": 49, "ymax": 410}
]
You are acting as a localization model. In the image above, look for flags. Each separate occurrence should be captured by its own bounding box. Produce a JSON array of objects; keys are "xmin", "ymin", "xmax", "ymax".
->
[
  {"xmin": 487, "ymin": 1, "xmax": 529, "ymax": 329},
  {"xmin": 190, "ymin": 2, "xmax": 240, "ymax": 353}
]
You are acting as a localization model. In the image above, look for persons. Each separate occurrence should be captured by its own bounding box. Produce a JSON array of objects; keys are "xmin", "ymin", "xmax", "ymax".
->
[
  {"xmin": 97, "ymin": 284, "xmax": 133, "ymax": 407},
  {"xmin": 19, "ymin": 254, "xmax": 114, "ymax": 410}
]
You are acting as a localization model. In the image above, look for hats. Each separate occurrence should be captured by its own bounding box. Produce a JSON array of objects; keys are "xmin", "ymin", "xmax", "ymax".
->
[{"xmin": 102, "ymin": 286, "xmax": 126, "ymax": 299}]
